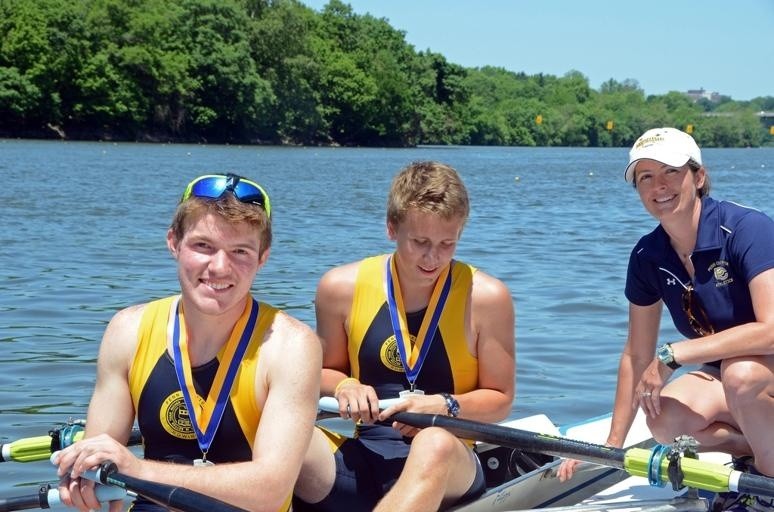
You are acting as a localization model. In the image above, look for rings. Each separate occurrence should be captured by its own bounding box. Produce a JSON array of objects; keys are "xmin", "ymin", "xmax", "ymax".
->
[{"xmin": 641, "ymin": 391, "xmax": 651, "ymax": 397}]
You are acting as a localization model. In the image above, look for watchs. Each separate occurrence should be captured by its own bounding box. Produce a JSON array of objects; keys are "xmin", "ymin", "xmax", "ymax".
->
[
  {"xmin": 440, "ymin": 393, "xmax": 459, "ymax": 417},
  {"xmin": 654, "ymin": 340, "xmax": 682, "ymax": 370}
]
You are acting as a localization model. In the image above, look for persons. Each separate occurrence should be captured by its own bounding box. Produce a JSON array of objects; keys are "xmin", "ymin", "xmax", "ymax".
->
[
  {"xmin": 51, "ymin": 172, "xmax": 324, "ymax": 512},
  {"xmin": 295, "ymin": 160, "xmax": 516, "ymax": 512},
  {"xmin": 554, "ymin": 128, "xmax": 774, "ymax": 511}
]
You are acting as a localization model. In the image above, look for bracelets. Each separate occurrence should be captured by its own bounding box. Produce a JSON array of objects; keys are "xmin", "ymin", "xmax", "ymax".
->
[{"xmin": 332, "ymin": 377, "xmax": 360, "ymax": 399}]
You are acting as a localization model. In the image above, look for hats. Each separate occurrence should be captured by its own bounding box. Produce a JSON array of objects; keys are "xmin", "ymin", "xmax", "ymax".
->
[{"xmin": 624, "ymin": 127, "xmax": 702, "ymax": 183}]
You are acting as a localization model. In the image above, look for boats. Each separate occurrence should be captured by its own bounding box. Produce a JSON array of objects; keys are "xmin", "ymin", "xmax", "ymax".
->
[{"xmin": 440, "ymin": 404, "xmax": 746, "ymax": 512}]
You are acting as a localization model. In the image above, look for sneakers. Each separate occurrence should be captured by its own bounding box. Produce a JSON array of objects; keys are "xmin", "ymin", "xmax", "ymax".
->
[{"xmin": 712, "ymin": 454, "xmax": 773, "ymax": 512}]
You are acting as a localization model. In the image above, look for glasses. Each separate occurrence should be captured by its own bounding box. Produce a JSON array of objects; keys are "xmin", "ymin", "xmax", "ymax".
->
[
  {"xmin": 184, "ymin": 174, "xmax": 272, "ymax": 217},
  {"xmin": 680, "ymin": 285, "xmax": 715, "ymax": 337}
]
